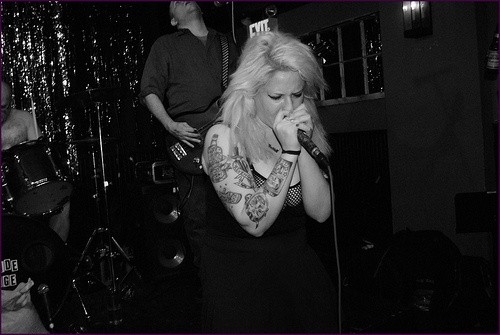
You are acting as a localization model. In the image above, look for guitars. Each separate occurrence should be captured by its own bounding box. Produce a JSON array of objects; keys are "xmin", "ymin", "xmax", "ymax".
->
[{"xmin": 164, "ymin": 96, "xmax": 221, "ymax": 174}]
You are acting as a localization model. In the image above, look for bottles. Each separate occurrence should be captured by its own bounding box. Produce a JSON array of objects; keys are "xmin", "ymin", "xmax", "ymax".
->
[{"xmin": 484, "ymin": 26, "xmax": 500, "ymax": 80}]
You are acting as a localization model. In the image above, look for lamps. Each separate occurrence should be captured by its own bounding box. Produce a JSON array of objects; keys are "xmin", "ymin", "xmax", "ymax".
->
[
  {"xmin": 402, "ymin": 0, "xmax": 433, "ymax": 39},
  {"xmin": 240, "ymin": 5, "xmax": 279, "ymax": 39}
]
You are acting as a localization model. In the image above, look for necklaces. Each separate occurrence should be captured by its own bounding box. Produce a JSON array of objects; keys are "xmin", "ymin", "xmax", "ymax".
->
[{"xmin": 269, "ymin": 144, "xmax": 279, "ymax": 153}]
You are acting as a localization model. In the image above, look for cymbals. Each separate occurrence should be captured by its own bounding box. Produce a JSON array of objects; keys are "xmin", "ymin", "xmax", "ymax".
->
[
  {"xmin": 57, "ymin": 87, "xmax": 120, "ymax": 106},
  {"xmin": 65, "ymin": 136, "xmax": 109, "ymax": 142}
]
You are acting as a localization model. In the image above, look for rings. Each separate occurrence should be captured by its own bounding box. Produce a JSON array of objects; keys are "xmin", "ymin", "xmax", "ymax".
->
[{"xmin": 296, "ymin": 124, "xmax": 299, "ymax": 126}]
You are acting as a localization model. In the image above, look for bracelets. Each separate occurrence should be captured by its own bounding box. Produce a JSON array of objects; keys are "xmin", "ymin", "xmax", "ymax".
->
[{"xmin": 282, "ymin": 149, "xmax": 301, "ymax": 155}]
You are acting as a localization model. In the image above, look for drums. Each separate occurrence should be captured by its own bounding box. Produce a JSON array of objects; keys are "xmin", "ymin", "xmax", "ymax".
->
[{"xmin": 0, "ymin": 139, "xmax": 78, "ymax": 218}]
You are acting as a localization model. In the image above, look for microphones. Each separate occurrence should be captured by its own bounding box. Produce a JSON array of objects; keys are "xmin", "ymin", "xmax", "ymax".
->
[{"xmin": 284, "ymin": 115, "xmax": 328, "ymax": 167}]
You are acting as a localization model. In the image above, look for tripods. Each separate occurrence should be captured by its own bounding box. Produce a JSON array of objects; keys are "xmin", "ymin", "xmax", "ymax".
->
[{"xmin": 41, "ymin": 99, "xmax": 174, "ymax": 335}]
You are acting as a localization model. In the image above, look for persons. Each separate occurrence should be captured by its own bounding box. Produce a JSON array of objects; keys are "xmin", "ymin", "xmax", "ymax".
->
[
  {"xmin": 1, "ymin": 82, "xmax": 69, "ymax": 245},
  {"xmin": 139, "ymin": 0, "xmax": 239, "ymax": 277},
  {"xmin": 1, "ymin": 282, "xmax": 49, "ymax": 333},
  {"xmin": 202, "ymin": 30, "xmax": 340, "ymax": 333}
]
